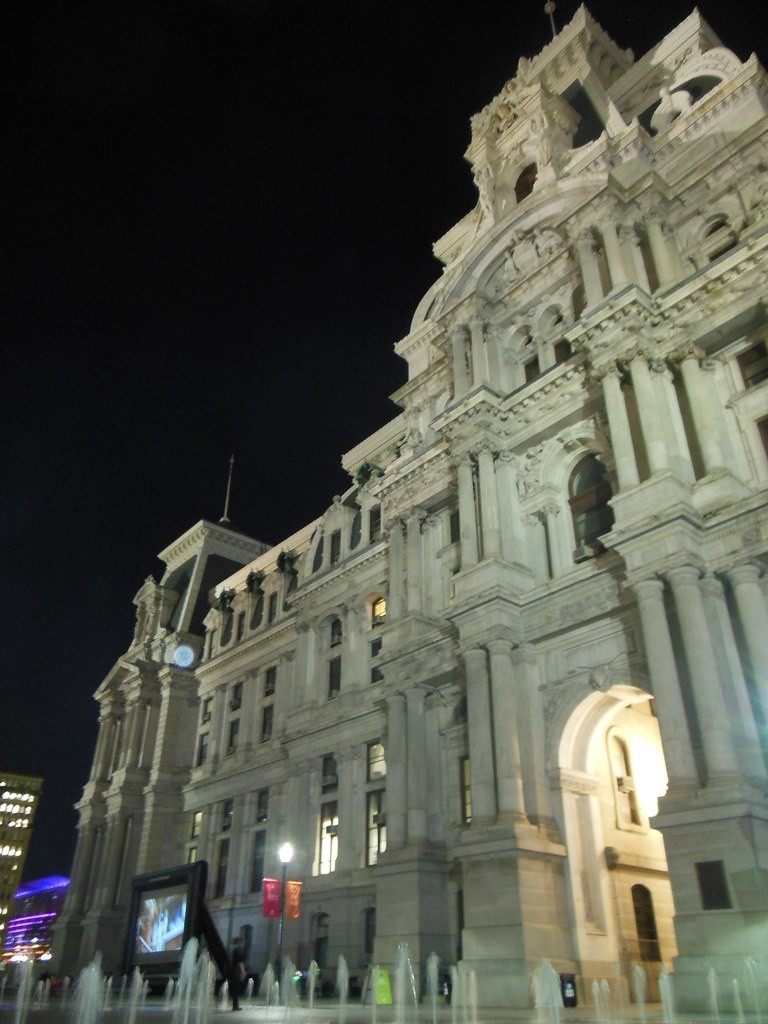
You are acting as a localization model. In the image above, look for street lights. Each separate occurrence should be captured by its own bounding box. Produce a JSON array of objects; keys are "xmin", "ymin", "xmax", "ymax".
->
[{"xmin": 271, "ymin": 841, "xmax": 294, "ymax": 1004}]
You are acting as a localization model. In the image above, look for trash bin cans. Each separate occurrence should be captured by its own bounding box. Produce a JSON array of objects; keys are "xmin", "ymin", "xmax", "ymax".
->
[
  {"xmin": 441, "ymin": 974, "xmax": 452, "ymax": 1004},
  {"xmin": 559, "ymin": 972, "xmax": 578, "ymax": 1009},
  {"xmin": 349, "ymin": 975, "xmax": 357, "ymax": 998}
]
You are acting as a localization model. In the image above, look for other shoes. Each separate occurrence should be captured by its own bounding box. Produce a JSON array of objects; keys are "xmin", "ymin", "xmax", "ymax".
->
[{"xmin": 232, "ymin": 1006, "xmax": 242, "ymax": 1011}]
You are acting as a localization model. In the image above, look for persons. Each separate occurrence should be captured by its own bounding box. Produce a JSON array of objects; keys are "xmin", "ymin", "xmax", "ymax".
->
[{"xmin": 214, "ymin": 924, "xmax": 253, "ymax": 1011}]
